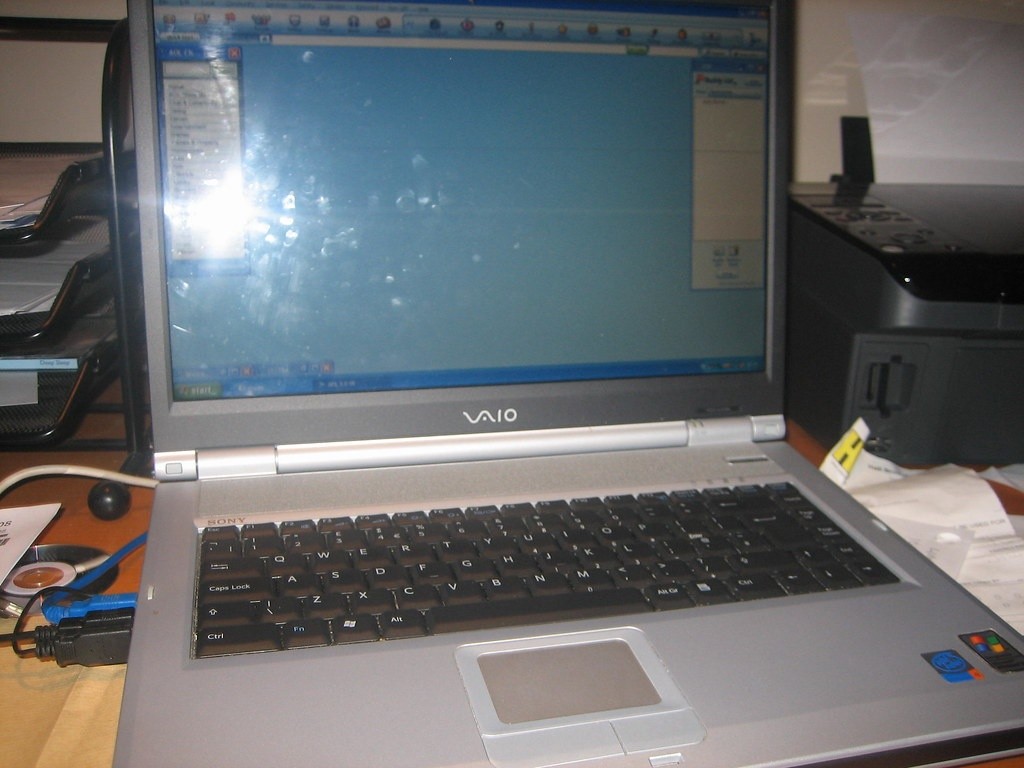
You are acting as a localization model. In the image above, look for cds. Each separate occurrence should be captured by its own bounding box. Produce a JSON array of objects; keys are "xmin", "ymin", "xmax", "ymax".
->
[{"xmin": 0, "ymin": 544, "xmax": 118, "ymax": 619}]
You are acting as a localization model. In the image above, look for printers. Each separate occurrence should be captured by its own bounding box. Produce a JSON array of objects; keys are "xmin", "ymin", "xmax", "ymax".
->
[{"xmin": 782, "ymin": 117, "xmax": 1024, "ymax": 469}]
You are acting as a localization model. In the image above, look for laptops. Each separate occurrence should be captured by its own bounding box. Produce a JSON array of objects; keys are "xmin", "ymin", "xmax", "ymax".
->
[{"xmin": 110, "ymin": 0, "xmax": 1024, "ymax": 768}]
